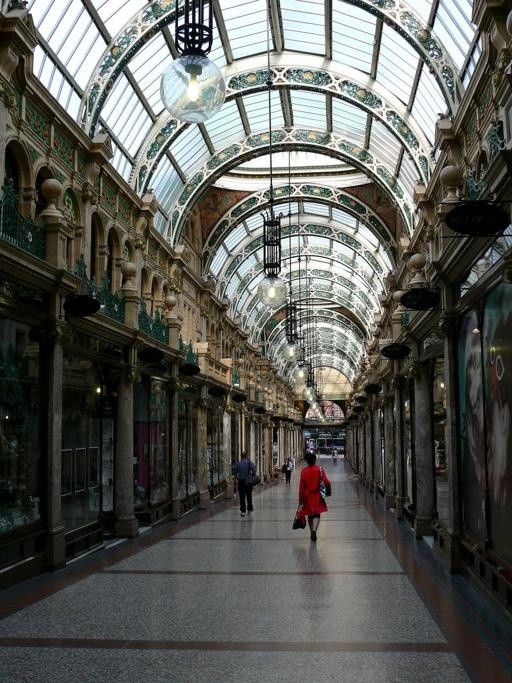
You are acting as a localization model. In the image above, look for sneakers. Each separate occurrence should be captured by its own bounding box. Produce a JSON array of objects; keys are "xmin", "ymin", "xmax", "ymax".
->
[
  {"xmin": 240, "ymin": 512, "xmax": 245, "ymax": 516},
  {"xmin": 311, "ymin": 529, "xmax": 316, "ymax": 542}
]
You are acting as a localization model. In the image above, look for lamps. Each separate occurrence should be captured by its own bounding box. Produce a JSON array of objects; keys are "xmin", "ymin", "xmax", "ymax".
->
[
  {"xmin": 160, "ymin": 0, "xmax": 226, "ymax": 125},
  {"xmin": 258, "ymin": 0, "xmax": 288, "ymax": 310}
]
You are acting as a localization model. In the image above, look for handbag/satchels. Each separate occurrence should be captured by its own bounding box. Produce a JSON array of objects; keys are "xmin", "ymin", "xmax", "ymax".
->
[
  {"xmin": 282, "ymin": 465, "xmax": 287, "ymax": 474},
  {"xmin": 292, "ymin": 508, "xmax": 306, "ymax": 529},
  {"xmin": 319, "ymin": 481, "xmax": 326, "ymax": 499},
  {"xmin": 246, "ymin": 473, "xmax": 261, "ymax": 487}
]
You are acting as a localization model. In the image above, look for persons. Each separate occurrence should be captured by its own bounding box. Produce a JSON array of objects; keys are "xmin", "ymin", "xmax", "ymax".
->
[
  {"xmin": 331, "ymin": 447, "xmax": 338, "ymax": 464},
  {"xmin": 298, "ymin": 452, "xmax": 331, "ymax": 542},
  {"xmin": 284, "ymin": 457, "xmax": 292, "ymax": 484},
  {"xmin": 231, "ymin": 451, "xmax": 256, "ymax": 516}
]
